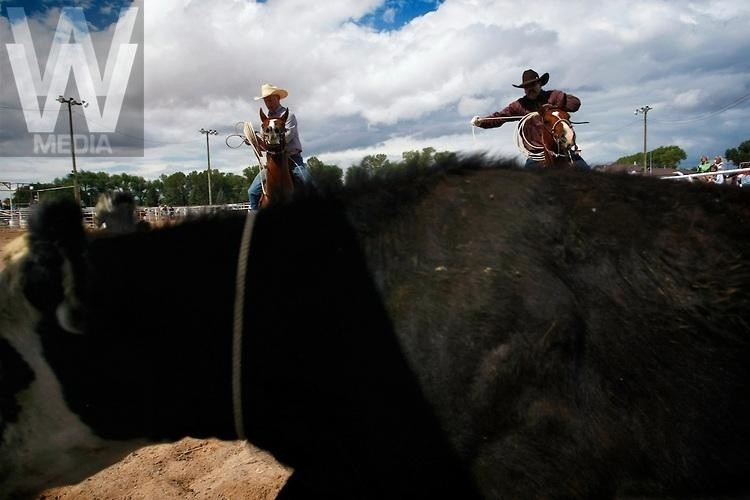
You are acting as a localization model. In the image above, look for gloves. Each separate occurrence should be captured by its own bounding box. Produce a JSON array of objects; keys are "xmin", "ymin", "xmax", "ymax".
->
[{"xmin": 470, "ymin": 115, "xmax": 481, "ymax": 127}]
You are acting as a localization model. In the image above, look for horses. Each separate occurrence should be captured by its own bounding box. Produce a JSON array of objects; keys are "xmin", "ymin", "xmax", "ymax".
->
[
  {"xmin": 530, "ymin": 92, "xmax": 590, "ymax": 172},
  {"xmin": 256, "ymin": 109, "xmax": 300, "ymax": 207}
]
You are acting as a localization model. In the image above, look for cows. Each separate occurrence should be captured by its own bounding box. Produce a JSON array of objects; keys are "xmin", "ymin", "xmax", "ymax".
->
[{"xmin": 0, "ymin": 145, "xmax": 750, "ymax": 500}]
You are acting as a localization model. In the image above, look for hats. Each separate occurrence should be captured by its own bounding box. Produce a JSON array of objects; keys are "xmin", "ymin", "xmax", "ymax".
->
[
  {"xmin": 253, "ymin": 83, "xmax": 288, "ymax": 101},
  {"xmin": 512, "ymin": 70, "xmax": 549, "ymax": 88}
]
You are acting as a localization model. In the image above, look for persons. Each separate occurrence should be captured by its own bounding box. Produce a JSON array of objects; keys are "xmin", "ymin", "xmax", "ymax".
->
[
  {"xmin": 697, "ymin": 156, "xmax": 714, "ymax": 173},
  {"xmin": 470, "ymin": 75, "xmax": 590, "ymax": 169},
  {"xmin": 707, "ymin": 165, "xmax": 725, "ymax": 185},
  {"xmin": 160, "ymin": 204, "xmax": 167, "ymax": 219},
  {"xmin": 244, "ymin": 90, "xmax": 324, "ymax": 209},
  {"xmin": 713, "ymin": 155, "xmax": 724, "ymax": 170},
  {"xmin": 168, "ymin": 205, "xmax": 176, "ymax": 217}
]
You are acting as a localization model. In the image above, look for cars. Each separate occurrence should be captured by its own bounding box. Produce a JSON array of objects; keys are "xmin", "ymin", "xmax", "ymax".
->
[
  {"xmin": 0, "ymin": 205, "xmax": 11, "ymax": 223},
  {"xmin": 12, "ymin": 202, "xmax": 30, "ymax": 215},
  {"xmin": 740, "ymin": 174, "xmax": 750, "ymax": 187}
]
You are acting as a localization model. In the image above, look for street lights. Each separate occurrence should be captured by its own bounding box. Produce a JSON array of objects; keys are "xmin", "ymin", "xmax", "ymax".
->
[
  {"xmin": 198, "ymin": 127, "xmax": 219, "ymax": 205},
  {"xmin": 632, "ymin": 104, "xmax": 654, "ymax": 175},
  {"xmin": 29, "ymin": 185, "xmax": 34, "ymax": 202},
  {"xmin": 54, "ymin": 94, "xmax": 89, "ymax": 205}
]
